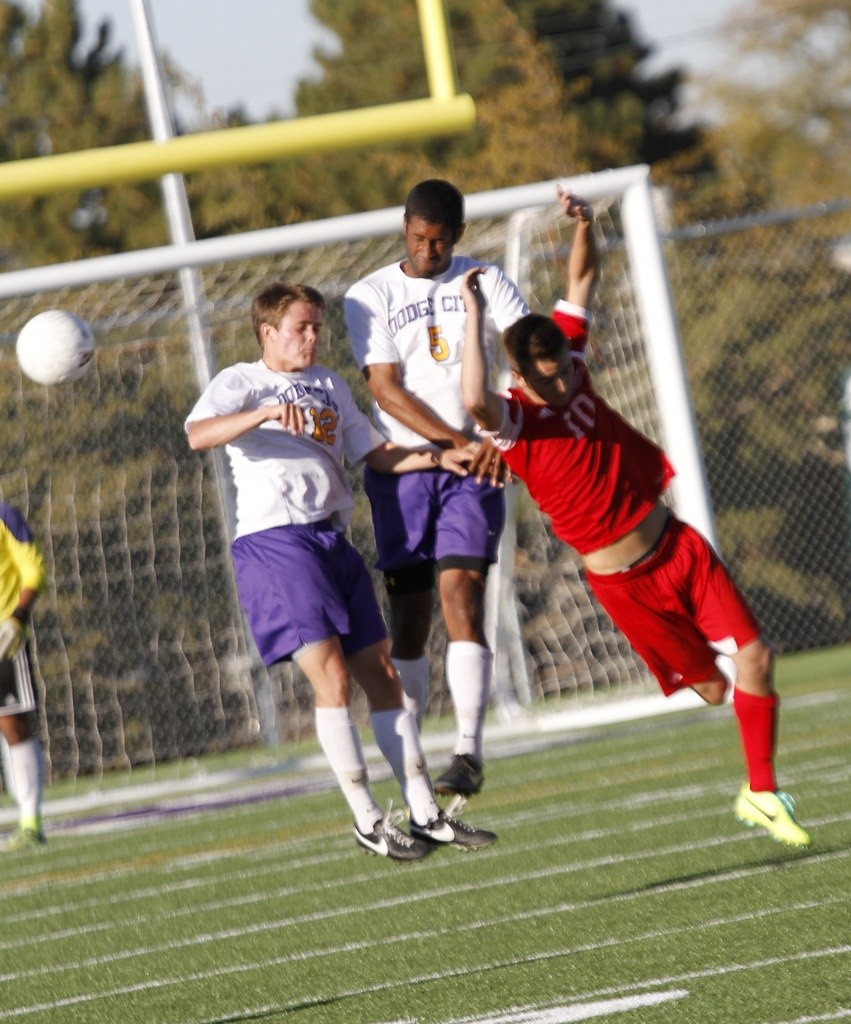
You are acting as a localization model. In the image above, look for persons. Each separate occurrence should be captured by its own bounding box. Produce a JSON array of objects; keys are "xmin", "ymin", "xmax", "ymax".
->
[
  {"xmin": 185, "ymin": 281, "xmax": 500, "ymax": 860},
  {"xmin": 460, "ymin": 184, "xmax": 812, "ymax": 852},
  {"xmin": 342, "ymin": 177, "xmax": 533, "ymax": 797},
  {"xmin": 0, "ymin": 501, "xmax": 54, "ymax": 857}
]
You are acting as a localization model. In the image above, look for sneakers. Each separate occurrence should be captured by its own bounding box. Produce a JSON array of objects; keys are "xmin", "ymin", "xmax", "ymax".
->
[
  {"xmin": 433, "ymin": 753, "xmax": 485, "ymax": 798},
  {"xmin": 408, "ymin": 810, "xmax": 498, "ymax": 851},
  {"xmin": 352, "ymin": 818, "xmax": 433, "ymax": 860},
  {"xmin": 733, "ymin": 783, "xmax": 812, "ymax": 850}
]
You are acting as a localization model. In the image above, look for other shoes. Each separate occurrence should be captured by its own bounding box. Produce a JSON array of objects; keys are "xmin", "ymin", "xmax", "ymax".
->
[{"xmin": 1, "ymin": 827, "xmax": 47, "ymax": 855}]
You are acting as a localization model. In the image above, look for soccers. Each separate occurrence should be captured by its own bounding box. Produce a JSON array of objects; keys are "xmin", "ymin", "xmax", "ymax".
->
[{"xmin": 14, "ymin": 309, "xmax": 95, "ymax": 386}]
